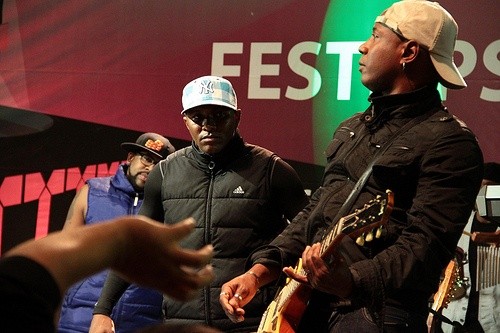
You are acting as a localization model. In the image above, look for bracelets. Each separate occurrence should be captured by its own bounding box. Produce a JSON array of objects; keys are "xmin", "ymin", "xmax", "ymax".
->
[{"xmin": 246, "ymin": 272, "xmax": 260, "ymax": 290}]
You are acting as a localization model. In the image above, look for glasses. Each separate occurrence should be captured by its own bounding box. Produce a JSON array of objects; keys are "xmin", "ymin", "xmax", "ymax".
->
[
  {"xmin": 136, "ymin": 152, "xmax": 159, "ymax": 167},
  {"xmin": 185, "ymin": 110, "xmax": 234, "ymax": 125}
]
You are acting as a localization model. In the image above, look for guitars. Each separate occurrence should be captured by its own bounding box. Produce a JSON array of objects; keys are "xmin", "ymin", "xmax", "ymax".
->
[
  {"xmin": 257, "ymin": 189, "xmax": 395, "ymax": 333},
  {"xmin": 426, "ymin": 259, "xmax": 463, "ymax": 333}
]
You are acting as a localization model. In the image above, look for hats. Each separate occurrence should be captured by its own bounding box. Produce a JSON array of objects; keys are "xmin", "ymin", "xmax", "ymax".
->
[
  {"xmin": 375, "ymin": 0, "xmax": 467, "ymax": 90},
  {"xmin": 180, "ymin": 75, "xmax": 237, "ymax": 115},
  {"xmin": 120, "ymin": 132, "xmax": 175, "ymax": 160}
]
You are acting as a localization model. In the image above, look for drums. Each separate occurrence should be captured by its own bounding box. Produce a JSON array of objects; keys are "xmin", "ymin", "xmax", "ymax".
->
[{"xmin": 447, "ymin": 246, "xmax": 468, "ymax": 302}]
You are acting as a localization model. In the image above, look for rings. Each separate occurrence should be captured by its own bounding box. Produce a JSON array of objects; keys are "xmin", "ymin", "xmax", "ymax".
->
[
  {"xmin": 220, "ymin": 293, "xmax": 229, "ymax": 299},
  {"xmin": 234, "ymin": 294, "xmax": 242, "ymax": 301},
  {"xmin": 307, "ymin": 270, "xmax": 312, "ymax": 277}
]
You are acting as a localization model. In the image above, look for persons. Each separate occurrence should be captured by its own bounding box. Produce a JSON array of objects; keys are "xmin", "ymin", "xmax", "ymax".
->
[
  {"xmin": 0, "ymin": 216, "xmax": 214, "ymax": 333},
  {"xmin": 220, "ymin": 0, "xmax": 483, "ymax": 333},
  {"xmin": 89, "ymin": 75, "xmax": 309, "ymax": 333},
  {"xmin": 441, "ymin": 162, "xmax": 500, "ymax": 333},
  {"xmin": 56, "ymin": 132, "xmax": 177, "ymax": 333}
]
